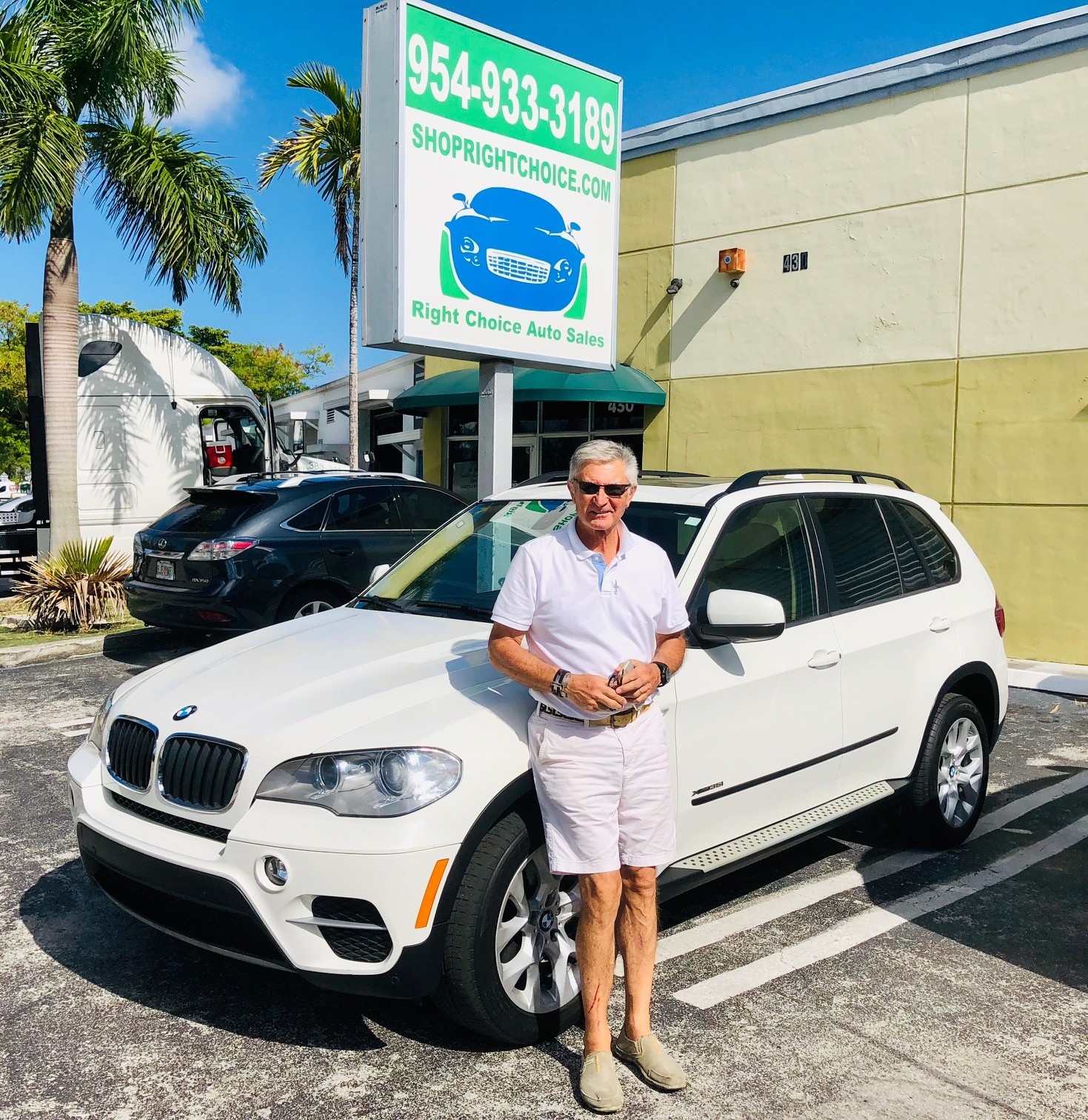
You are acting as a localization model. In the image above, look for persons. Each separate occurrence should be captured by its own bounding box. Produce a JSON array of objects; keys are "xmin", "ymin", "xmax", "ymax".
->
[{"xmin": 487, "ymin": 442, "xmax": 688, "ymax": 1112}]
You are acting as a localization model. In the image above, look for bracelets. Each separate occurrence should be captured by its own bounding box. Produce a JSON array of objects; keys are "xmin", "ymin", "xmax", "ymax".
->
[{"xmin": 551, "ymin": 669, "xmax": 571, "ymax": 698}]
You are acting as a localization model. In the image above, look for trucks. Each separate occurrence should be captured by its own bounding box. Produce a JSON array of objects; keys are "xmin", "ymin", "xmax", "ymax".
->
[{"xmin": 24, "ymin": 313, "xmax": 374, "ymax": 588}]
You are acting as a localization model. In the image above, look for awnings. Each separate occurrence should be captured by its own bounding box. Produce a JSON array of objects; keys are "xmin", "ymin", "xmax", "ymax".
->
[{"xmin": 393, "ymin": 362, "xmax": 667, "ymax": 417}]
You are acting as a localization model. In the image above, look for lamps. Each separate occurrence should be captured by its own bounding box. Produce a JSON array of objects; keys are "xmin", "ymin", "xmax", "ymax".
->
[{"xmin": 666, "ymin": 278, "xmax": 682, "ymax": 294}]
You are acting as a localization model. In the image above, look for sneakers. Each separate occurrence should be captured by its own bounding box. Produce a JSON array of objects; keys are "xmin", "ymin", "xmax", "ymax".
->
[
  {"xmin": 615, "ymin": 1024, "xmax": 687, "ymax": 1091},
  {"xmin": 580, "ymin": 1051, "xmax": 624, "ymax": 1112}
]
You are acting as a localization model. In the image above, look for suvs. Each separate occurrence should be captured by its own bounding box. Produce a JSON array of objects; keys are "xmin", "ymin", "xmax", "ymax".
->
[
  {"xmin": 123, "ymin": 467, "xmax": 534, "ymax": 637},
  {"xmin": 66, "ymin": 466, "xmax": 1007, "ymax": 1045}
]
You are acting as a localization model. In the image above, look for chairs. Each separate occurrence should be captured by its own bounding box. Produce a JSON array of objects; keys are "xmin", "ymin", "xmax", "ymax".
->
[{"xmin": 704, "ymin": 523, "xmax": 790, "ymax": 620}]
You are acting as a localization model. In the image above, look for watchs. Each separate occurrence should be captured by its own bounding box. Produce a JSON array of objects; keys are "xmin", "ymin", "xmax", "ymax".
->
[{"xmin": 651, "ymin": 661, "xmax": 671, "ymax": 687}]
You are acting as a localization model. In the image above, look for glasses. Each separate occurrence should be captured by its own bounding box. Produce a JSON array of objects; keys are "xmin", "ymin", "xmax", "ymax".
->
[{"xmin": 572, "ymin": 477, "xmax": 631, "ymax": 497}]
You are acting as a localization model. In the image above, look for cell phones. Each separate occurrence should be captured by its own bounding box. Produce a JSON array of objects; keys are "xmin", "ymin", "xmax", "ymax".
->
[{"xmin": 617, "ymin": 659, "xmax": 635, "ymax": 679}]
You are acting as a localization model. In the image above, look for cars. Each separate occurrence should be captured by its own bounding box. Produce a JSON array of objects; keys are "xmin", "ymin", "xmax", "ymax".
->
[{"xmin": 0, "ymin": 479, "xmax": 34, "ymax": 526}]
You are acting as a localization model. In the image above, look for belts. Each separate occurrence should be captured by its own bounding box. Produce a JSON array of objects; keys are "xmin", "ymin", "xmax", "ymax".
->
[{"xmin": 536, "ymin": 700, "xmax": 652, "ymax": 728}]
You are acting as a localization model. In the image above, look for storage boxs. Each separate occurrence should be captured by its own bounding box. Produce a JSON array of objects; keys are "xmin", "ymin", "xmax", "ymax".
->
[{"xmin": 203, "ymin": 441, "xmax": 232, "ymax": 468}]
